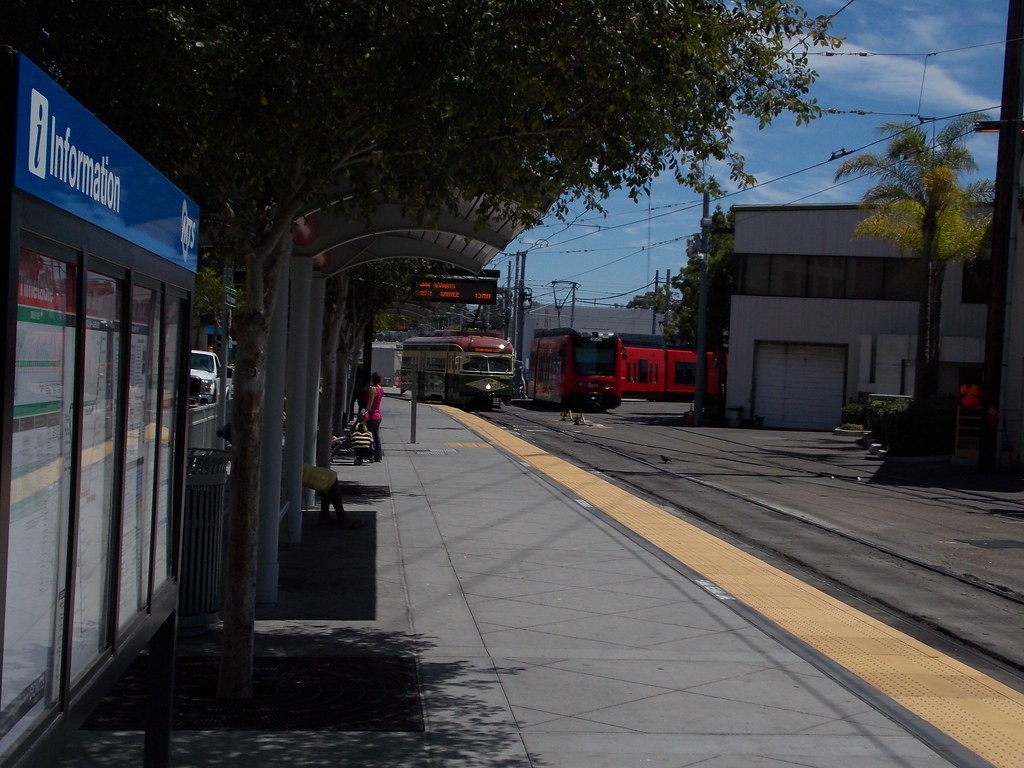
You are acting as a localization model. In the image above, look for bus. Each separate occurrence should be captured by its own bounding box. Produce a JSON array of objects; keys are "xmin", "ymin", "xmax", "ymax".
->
[
  {"xmin": 399, "ymin": 329, "xmax": 517, "ymax": 412},
  {"xmin": 514, "ymin": 327, "xmax": 728, "ymax": 413}
]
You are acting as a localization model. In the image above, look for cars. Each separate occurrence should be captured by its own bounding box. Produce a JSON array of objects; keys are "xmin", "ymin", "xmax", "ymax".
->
[{"xmin": 188, "ymin": 349, "xmax": 234, "ymax": 412}]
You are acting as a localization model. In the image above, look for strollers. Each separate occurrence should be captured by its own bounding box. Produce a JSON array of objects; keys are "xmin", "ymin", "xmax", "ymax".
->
[{"xmin": 329, "ymin": 412, "xmax": 362, "ymax": 465}]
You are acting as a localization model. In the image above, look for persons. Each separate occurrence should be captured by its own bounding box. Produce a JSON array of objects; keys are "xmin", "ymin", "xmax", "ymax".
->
[
  {"xmin": 217, "ymin": 423, "xmax": 368, "ymax": 529},
  {"xmin": 363, "ymin": 372, "xmax": 383, "ymax": 462},
  {"xmin": 959, "ymin": 375, "xmax": 984, "ymax": 411}
]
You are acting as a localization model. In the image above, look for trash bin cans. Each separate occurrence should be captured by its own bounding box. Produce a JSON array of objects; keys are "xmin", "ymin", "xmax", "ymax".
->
[
  {"xmin": 171, "ymin": 446, "xmax": 234, "ymax": 639},
  {"xmin": 727, "ymin": 406, "xmax": 744, "ymax": 427}
]
